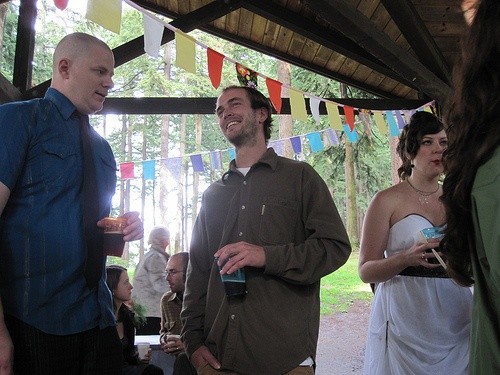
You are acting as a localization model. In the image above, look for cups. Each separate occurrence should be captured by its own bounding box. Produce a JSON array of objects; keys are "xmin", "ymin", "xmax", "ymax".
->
[
  {"xmin": 218, "ymin": 257, "xmax": 249, "ymax": 301},
  {"xmin": 421, "ymin": 224, "xmax": 446, "ymax": 266},
  {"xmin": 136, "ymin": 340, "xmax": 152, "ymax": 360},
  {"xmin": 166, "ymin": 334, "xmax": 180, "ymax": 352},
  {"xmin": 101, "ymin": 217, "xmax": 129, "ymax": 257}
]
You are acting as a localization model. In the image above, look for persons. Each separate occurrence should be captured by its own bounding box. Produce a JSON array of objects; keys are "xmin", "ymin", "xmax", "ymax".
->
[
  {"xmin": 358, "ymin": 110, "xmax": 474, "ymax": 375},
  {"xmin": 438, "ymin": 0, "xmax": 500, "ymax": 375},
  {"xmin": 180, "ymin": 85, "xmax": 353, "ymax": 375},
  {"xmin": 105, "ymin": 265, "xmax": 164, "ymax": 375},
  {"xmin": 131, "ymin": 227, "xmax": 173, "ymax": 335},
  {"xmin": 159, "ymin": 252, "xmax": 197, "ymax": 375},
  {"xmin": 0, "ymin": 32, "xmax": 144, "ymax": 375}
]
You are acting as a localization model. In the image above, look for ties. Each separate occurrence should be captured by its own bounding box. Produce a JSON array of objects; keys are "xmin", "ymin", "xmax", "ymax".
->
[{"xmin": 77, "ymin": 114, "xmax": 104, "ymax": 287}]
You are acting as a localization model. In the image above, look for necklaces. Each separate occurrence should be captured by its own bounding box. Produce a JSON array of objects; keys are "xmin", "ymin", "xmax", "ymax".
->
[{"xmin": 406, "ymin": 176, "xmax": 440, "ymax": 205}]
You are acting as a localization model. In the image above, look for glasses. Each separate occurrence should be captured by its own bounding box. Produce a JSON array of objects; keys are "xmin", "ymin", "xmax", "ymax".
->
[{"xmin": 162, "ymin": 269, "xmax": 187, "ymax": 277}]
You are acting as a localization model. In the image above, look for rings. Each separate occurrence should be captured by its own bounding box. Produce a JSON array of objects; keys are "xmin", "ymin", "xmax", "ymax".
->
[
  {"xmin": 175, "ymin": 347, "xmax": 179, "ymax": 350},
  {"xmin": 421, "ymin": 251, "xmax": 429, "ymax": 263}
]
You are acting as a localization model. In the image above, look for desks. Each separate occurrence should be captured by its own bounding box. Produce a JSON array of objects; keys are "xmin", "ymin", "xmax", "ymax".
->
[{"xmin": 134, "ymin": 333, "xmax": 177, "ymax": 375}]
